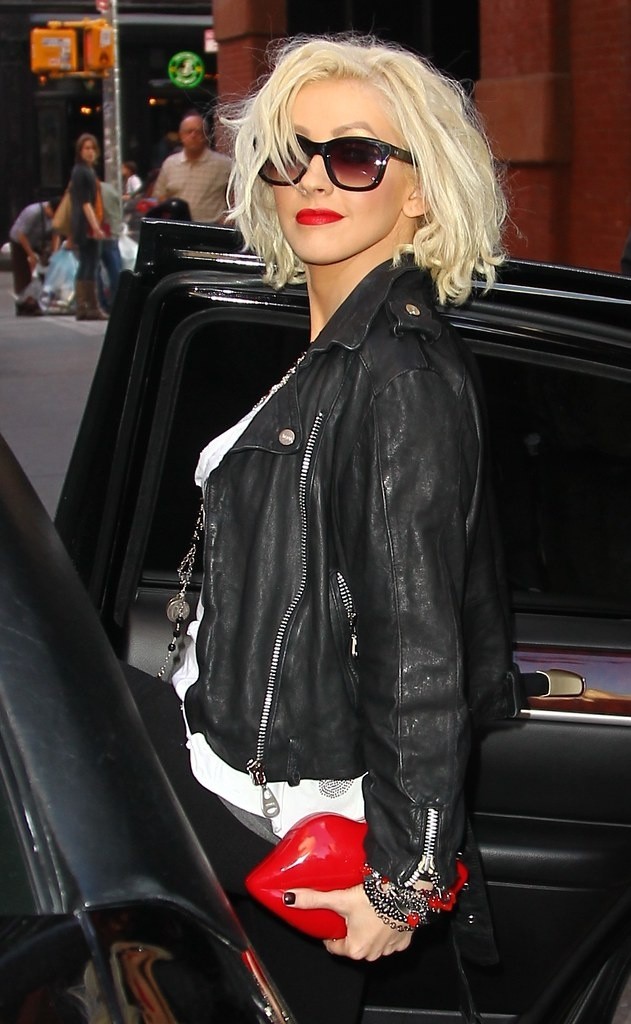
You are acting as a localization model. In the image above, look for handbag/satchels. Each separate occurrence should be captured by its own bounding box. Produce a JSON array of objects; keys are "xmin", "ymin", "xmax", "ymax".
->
[
  {"xmin": 245, "ymin": 812, "xmax": 466, "ymax": 939},
  {"xmin": 47, "ymin": 189, "xmax": 73, "ymax": 237}
]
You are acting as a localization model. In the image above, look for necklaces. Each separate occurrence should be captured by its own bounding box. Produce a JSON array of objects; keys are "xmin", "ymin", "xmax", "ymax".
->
[{"xmin": 153, "ymin": 347, "xmax": 308, "ymax": 682}]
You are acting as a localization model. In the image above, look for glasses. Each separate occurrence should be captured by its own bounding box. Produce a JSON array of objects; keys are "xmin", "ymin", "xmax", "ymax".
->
[{"xmin": 253, "ymin": 133, "xmax": 419, "ymax": 192}]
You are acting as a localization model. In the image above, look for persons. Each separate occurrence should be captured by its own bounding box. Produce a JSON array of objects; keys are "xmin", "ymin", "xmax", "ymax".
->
[
  {"xmin": 171, "ymin": 33, "xmax": 529, "ymax": 1023},
  {"xmin": 145, "ymin": 114, "xmax": 238, "ymax": 229},
  {"xmin": 0, "ymin": 133, "xmax": 142, "ymax": 321}
]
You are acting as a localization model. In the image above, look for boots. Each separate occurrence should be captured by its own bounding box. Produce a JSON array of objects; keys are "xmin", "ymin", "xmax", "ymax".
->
[{"xmin": 74, "ymin": 278, "xmax": 109, "ymax": 321}]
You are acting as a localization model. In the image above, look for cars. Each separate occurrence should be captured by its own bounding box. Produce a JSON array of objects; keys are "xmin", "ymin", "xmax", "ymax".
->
[{"xmin": 0, "ymin": 220, "xmax": 631, "ymax": 1024}]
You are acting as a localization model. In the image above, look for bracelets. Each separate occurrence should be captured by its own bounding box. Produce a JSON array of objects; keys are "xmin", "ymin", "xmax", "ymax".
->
[{"xmin": 362, "ymin": 872, "xmax": 430, "ymax": 933}]
